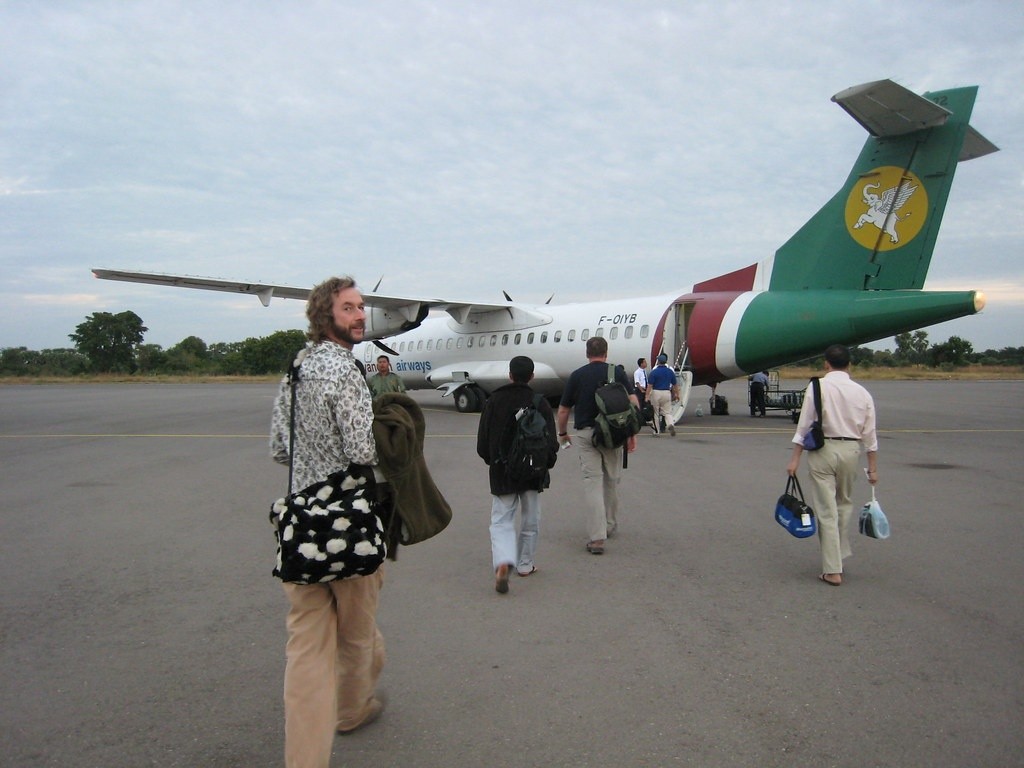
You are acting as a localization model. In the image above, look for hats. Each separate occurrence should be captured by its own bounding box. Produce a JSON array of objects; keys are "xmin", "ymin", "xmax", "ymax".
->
[{"xmin": 656, "ymin": 355, "xmax": 667, "ymax": 362}]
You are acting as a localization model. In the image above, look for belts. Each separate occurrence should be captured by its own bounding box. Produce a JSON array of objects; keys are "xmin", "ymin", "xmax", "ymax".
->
[{"xmin": 824, "ymin": 437, "xmax": 857, "ymax": 440}]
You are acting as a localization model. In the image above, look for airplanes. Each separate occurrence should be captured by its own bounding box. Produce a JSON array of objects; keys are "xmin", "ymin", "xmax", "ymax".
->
[{"xmin": 90, "ymin": 77, "xmax": 1001, "ymax": 413}]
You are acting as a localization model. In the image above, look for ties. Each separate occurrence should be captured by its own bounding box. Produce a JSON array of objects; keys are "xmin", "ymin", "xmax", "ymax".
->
[{"xmin": 643, "ymin": 371, "xmax": 647, "ymax": 387}]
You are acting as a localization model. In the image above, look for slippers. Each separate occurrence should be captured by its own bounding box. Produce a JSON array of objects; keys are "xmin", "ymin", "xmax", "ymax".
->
[
  {"xmin": 518, "ymin": 566, "xmax": 535, "ymax": 576},
  {"xmin": 496, "ymin": 565, "xmax": 509, "ymax": 593}
]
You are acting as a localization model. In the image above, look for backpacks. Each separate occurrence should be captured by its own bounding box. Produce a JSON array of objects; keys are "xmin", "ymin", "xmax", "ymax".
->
[
  {"xmin": 591, "ymin": 363, "xmax": 640, "ymax": 449},
  {"xmin": 499, "ymin": 393, "xmax": 552, "ymax": 481},
  {"xmin": 642, "ymin": 401, "xmax": 654, "ymax": 420}
]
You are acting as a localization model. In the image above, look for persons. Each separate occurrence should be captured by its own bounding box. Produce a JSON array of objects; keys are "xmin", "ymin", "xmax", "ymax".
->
[
  {"xmin": 748, "ymin": 371, "xmax": 770, "ymax": 418},
  {"xmin": 557, "ymin": 336, "xmax": 641, "ymax": 554},
  {"xmin": 477, "ymin": 356, "xmax": 561, "ymax": 593},
  {"xmin": 616, "ymin": 354, "xmax": 680, "ymax": 436},
  {"xmin": 365, "ymin": 355, "xmax": 406, "ymax": 412},
  {"xmin": 269, "ymin": 275, "xmax": 386, "ymax": 768},
  {"xmin": 786, "ymin": 344, "xmax": 879, "ymax": 586}
]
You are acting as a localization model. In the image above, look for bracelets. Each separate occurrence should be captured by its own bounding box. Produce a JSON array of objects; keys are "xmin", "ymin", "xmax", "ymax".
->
[
  {"xmin": 867, "ymin": 470, "xmax": 877, "ymax": 473},
  {"xmin": 557, "ymin": 431, "xmax": 567, "ymax": 436}
]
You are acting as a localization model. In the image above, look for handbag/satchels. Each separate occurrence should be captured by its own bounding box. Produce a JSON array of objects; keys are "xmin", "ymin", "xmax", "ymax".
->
[
  {"xmin": 269, "ymin": 468, "xmax": 386, "ymax": 585},
  {"xmin": 858, "ymin": 468, "xmax": 889, "ymax": 539},
  {"xmin": 802, "ymin": 377, "xmax": 825, "ymax": 451},
  {"xmin": 775, "ymin": 473, "xmax": 816, "ymax": 538}
]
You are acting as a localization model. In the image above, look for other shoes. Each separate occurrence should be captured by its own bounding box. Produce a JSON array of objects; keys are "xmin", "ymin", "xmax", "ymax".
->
[
  {"xmin": 587, "ymin": 540, "xmax": 603, "ymax": 553},
  {"xmin": 337, "ymin": 696, "xmax": 382, "ymax": 735}
]
